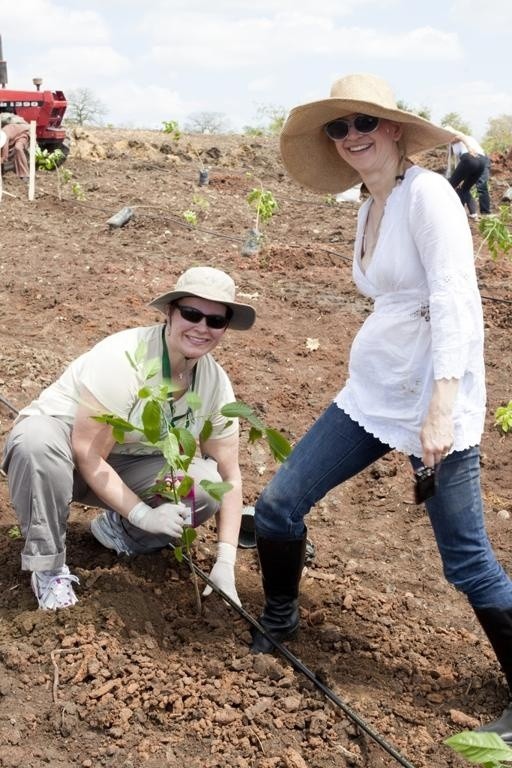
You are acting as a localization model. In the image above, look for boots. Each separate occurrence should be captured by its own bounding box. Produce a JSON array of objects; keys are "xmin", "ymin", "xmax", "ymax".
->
[
  {"xmin": 470, "ymin": 606, "xmax": 512, "ymax": 691},
  {"xmin": 249, "ymin": 525, "xmax": 307, "ymax": 655}
]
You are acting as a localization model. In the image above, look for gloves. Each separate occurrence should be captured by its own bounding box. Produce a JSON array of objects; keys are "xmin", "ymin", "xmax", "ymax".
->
[
  {"xmin": 127, "ymin": 501, "xmax": 187, "ymax": 540},
  {"xmin": 201, "ymin": 543, "xmax": 243, "ymax": 610}
]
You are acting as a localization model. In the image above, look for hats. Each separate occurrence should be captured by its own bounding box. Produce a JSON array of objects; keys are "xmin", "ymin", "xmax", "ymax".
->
[
  {"xmin": 280, "ymin": 73, "xmax": 458, "ymax": 194},
  {"xmin": 147, "ymin": 266, "xmax": 257, "ymax": 331}
]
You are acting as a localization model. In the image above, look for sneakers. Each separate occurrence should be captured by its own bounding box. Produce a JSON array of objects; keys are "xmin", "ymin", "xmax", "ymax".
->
[
  {"xmin": 89, "ymin": 509, "xmax": 131, "ymax": 555},
  {"xmin": 30, "ymin": 564, "xmax": 79, "ymax": 610}
]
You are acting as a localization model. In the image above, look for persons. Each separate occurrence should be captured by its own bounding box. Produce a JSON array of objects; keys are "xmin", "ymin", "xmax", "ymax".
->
[
  {"xmin": 248, "ymin": 71, "xmax": 511, "ymax": 744},
  {"xmin": 444, "ymin": 123, "xmax": 487, "ymax": 219},
  {"xmin": 458, "ymin": 137, "xmax": 492, "ymax": 214},
  {"xmin": 1, "ymin": 124, "xmax": 31, "ymax": 179},
  {"xmin": 1, "ymin": 266, "xmax": 257, "ymax": 611}
]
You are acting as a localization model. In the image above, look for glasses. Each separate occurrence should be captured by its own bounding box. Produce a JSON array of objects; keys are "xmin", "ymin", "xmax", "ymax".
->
[
  {"xmin": 174, "ymin": 301, "xmax": 229, "ymax": 329},
  {"xmin": 323, "ymin": 115, "xmax": 381, "ymax": 143}
]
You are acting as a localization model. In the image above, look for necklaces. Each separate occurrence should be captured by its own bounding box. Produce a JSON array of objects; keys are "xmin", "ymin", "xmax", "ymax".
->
[{"xmin": 169, "ymin": 356, "xmax": 195, "ymax": 380}]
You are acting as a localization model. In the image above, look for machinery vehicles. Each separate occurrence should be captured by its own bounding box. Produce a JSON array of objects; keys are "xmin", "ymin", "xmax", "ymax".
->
[{"xmin": 0, "ymin": 33, "xmax": 67, "ymax": 170}]
